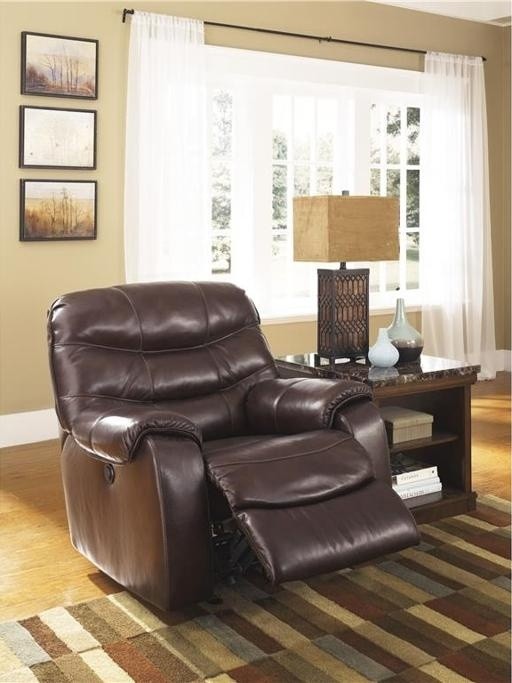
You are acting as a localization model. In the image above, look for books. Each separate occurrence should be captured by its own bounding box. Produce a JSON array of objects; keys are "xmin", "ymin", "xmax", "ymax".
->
[
  {"xmin": 378, "ymin": 405, "xmax": 434, "ymax": 429},
  {"xmin": 391, "ymin": 476, "xmax": 441, "ymax": 490},
  {"xmin": 390, "ymin": 452, "xmax": 438, "ymax": 483},
  {"xmin": 384, "ymin": 423, "xmax": 432, "ymax": 443},
  {"xmin": 396, "ymin": 482, "xmax": 442, "ymax": 499}
]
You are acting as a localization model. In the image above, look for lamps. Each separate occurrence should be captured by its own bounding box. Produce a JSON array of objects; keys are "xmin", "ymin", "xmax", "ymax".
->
[{"xmin": 288, "ymin": 184, "xmax": 406, "ymax": 370}]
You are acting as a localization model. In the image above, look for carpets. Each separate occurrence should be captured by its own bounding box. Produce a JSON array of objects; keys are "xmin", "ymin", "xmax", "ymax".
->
[{"xmin": 2, "ymin": 492, "xmax": 511, "ymax": 683}]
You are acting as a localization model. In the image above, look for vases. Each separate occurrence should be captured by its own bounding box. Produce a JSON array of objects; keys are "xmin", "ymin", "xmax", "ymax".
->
[
  {"xmin": 368, "ymin": 326, "xmax": 402, "ymax": 367},
  {"xmin": 385, "ymin": 295, "xmax": 425, "ymax": 362}
]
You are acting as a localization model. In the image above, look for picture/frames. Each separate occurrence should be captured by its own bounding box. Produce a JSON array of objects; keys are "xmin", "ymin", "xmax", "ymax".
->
[
  {"xmin": 18, "ymin": 29, "xmax": 101, "ymax": 102},
  {"xmin": 17, "ymin": 102, "xmax": 99, "ymax": 171},
  {"xmin": 17, "ymin": 177, "xmax": 99, "ymax": 243}
]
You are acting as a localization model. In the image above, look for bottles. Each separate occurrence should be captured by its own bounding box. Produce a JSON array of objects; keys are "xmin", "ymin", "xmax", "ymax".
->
[{"xmin": 368, "ymin": 297, "xmax": 424, "ymax": 367}]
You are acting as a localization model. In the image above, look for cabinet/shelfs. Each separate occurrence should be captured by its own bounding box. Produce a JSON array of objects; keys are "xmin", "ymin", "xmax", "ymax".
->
[{"xmin": 271, "ymin": 349, "xmax": 486, "ymax": 525}]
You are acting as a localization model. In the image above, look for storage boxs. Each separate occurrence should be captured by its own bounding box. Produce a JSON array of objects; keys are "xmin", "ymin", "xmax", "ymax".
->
[{"xmin": 376, "ymin": 404, "xmax": 435, "ymax": 446}]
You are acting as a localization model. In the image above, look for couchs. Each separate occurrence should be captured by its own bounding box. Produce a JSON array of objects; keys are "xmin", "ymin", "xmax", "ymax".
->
[{"xmin": 45, "ymin": 278, "xmax": 431, "ymax": 617}]
